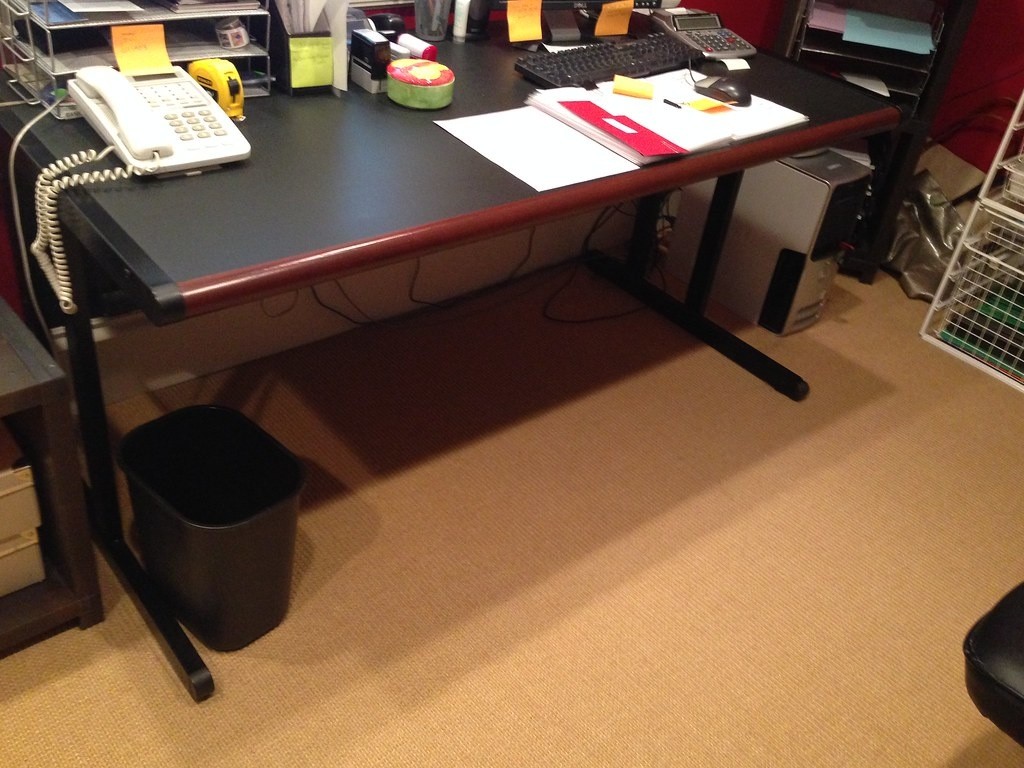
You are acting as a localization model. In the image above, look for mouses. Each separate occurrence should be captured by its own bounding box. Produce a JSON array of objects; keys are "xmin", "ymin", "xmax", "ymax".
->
[{"xmin": 694, "ymin": 76, "xmax": 752, "ymax": 106}]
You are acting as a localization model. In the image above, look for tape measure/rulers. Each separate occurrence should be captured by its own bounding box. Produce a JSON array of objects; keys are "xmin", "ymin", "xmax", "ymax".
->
[{"xmin": 188, "ymin": 59, "xmax": 246, "ymax": 123}]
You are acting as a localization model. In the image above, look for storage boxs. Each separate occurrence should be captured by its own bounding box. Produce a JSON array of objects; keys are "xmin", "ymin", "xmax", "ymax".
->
[
  {"xmin": 912, "ymin": 137, "xmax": 1007, "ymax": 250},
  {"xmin": 0, "ymin": 453, "xmax": 47, "ymax": 597}
]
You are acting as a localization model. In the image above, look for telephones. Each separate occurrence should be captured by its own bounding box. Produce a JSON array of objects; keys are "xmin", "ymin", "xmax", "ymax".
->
[{"xmin": 66, "ymin": 66, "xmax": 253, "ymax": 178}]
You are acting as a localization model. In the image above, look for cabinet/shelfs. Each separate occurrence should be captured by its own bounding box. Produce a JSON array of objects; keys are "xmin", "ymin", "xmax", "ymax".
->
[
  {"xmin": 777, "ymin": 0, "xmax": 976, "ymax": 285},
  {"xmin": 2, "ymin": 1, "xmax": 272, "ymax": 121},
  {"xmin": 0, "ymin": 297, "xmax": 103, "ymax": 657},
  {"xmin": 921, "ymin": 86, "xmax": 1024, "ymax": 394}
]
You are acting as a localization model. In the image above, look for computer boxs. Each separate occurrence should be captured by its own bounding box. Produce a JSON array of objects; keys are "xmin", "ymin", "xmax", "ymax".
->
[{"xmin": 664, "ymin": 144, "xmax": 873, "ymax": 336}]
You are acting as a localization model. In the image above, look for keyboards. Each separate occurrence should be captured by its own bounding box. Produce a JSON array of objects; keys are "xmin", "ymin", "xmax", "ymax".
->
[{"xmin": 514, "ymin": 30, "xmax": 706, "ymax": 89}]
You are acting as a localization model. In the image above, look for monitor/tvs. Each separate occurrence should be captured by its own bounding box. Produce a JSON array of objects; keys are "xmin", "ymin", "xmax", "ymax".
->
[{"xmin": 486, "ymin": 0, "xmax": 662, "ymax": 53}]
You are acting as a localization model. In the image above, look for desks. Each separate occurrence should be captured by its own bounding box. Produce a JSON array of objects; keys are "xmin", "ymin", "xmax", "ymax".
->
[{"xmin": 1, "ymin": 21, "xmax": 903, "ymax": 702}]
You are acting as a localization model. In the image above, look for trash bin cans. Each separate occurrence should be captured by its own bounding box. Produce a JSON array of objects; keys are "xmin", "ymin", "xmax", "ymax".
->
[{"xmin": 114, "ymin": 403, "xmax": 308, "ymax": 654}]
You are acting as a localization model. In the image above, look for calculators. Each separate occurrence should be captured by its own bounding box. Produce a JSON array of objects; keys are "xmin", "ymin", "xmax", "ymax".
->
[{"xmin": 648, "ymin": 8, "xmax": 759, "ymax": 59}]
